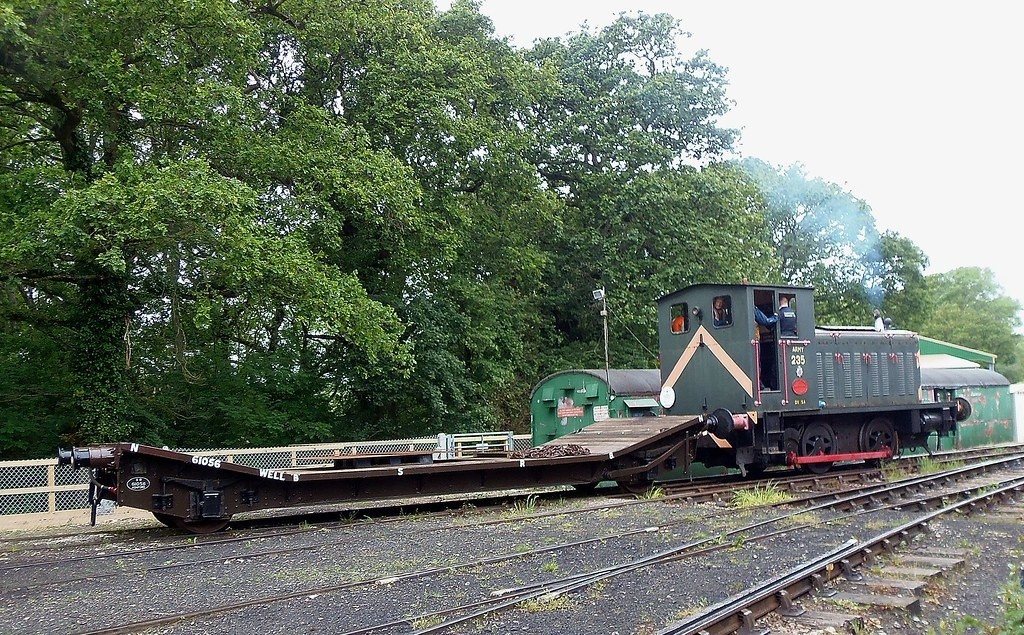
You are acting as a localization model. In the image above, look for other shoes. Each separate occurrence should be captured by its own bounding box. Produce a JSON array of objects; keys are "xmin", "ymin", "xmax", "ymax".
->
[{"xmin": 764, "ymin": 388, "xmax": 770, "ymax": 391}]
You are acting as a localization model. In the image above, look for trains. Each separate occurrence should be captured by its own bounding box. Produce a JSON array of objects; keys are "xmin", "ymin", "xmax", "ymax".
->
[{"xmin": 55, "ymin": 279, "xmax": 973, "ymax": 536}]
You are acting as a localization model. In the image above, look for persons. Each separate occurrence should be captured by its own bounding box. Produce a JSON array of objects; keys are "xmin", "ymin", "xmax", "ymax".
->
[
  {"xmin": 713, "ymin": 296, "xmax": 732, "ymax": 326},
  {"xmin": 754, "ymin": 297, "xmax": 797, "ymax": 390},
  {"xmin": 672, "ymin": 308, "xmax": 684, "ymax": 332}
]
